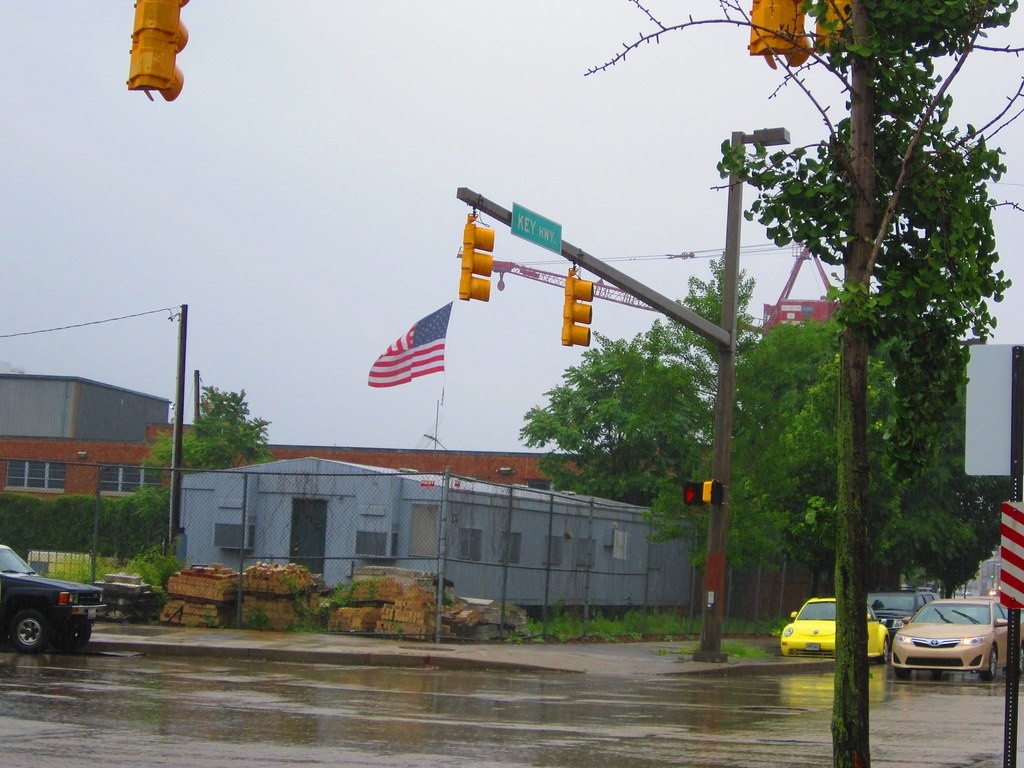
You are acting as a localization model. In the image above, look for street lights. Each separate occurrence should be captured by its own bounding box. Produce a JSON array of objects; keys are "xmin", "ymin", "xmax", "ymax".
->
[{"xmin": 692, "ymin": 128, "xmax": 791, "ymax": 663}]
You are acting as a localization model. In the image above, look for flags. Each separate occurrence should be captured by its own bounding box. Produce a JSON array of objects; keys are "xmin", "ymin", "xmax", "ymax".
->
[{"xmin": 368, "ymin": 299, "xmax": 453, "ymax": 388}]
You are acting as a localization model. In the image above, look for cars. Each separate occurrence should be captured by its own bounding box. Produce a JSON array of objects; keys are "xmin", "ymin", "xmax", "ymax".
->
[
  {"xmin": 889, "ymin": 597, "xmax": 1024, "ymax": 681},
  {"xmin": 0, "ymin": 545, "xmax": 108, "ymax": 654},
  {"xmin": 866, "ymin": 587, "xmax": 942, "ymax": 650},
  {"xmin": 781, "ymin": 597, "xmax": 890, "ymax": 665}
]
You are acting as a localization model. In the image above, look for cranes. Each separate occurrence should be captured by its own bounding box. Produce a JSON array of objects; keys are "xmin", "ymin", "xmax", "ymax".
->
[{"xmin": 457, "ymin": 238, "xmax": 841, "ymax": 338}]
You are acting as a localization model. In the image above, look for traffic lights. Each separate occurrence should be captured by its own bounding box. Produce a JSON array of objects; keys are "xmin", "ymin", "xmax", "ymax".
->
[
  {"xmin": 460, "ymin": 227, "xmax": 495, "ymax": 302},
  {"xmin": 561, "ymin": 280, "xmax": 593, "ymax": 347},
  {"xmin": 683, "ymin": 483, "xmax": 723, "ymax": 504},
  {"xmin": 749, "ymin": 0, "xmax": 813, "ymax": 67},
  {"xmin": 129, "ymin": 0, "xmax": 190, "ymax": 102},
  {"xmin": 991, "ymin": 574, "xmax": 994, "ymax": 579}
]
